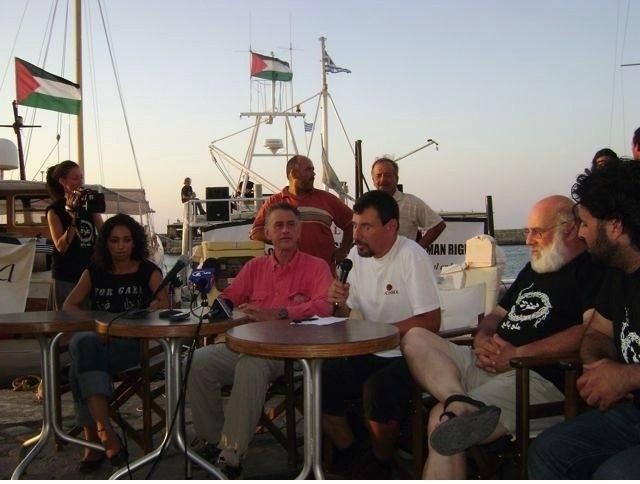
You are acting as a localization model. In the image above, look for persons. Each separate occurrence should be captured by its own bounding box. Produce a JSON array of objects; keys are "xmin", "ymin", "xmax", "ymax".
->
[
  {"xmin": 238, "ymin": 176, "xmax": 254, "ymax": 198},
  {"xmin": 61, "ymin": 213, "xmax": 170, "ymax": 472},
  {"xmin": 181, "ymin": 177, "xmax": 206, "ymax": 215},
  {"xmin": 632, "ymin": 129, "xmax": 639, "ymax": 160},
  {"xmin": 400, "ymin": 195, "xmax": 610, "ymax": 478},
  {"xmin": 249, "ymin": 156, "xmax": 355, "ymax": 320},
  {"xmin": 181, "ymin": 204, "xmax": 336, "ymax": 480},
  {"xmin": 45, "ymin": 161, "xmax": 104, "ymax": 313},
  {"xmin": 592, "ymin": 149, "xmax": 617, "ymax": 166},
  {"xmin": 327, "ymin": 190, "xmax": 440, "ymax": 480},
  {"xmin": 371, "ymin": 158, "xmax": 446, "ymax": 248},
  {"xmin": 524, "ymin": 159, "xmax": 638, "ymax": 479},
  {"xmin": 0, "ymin": 225, "xmax": 20, "ymax": 245}
]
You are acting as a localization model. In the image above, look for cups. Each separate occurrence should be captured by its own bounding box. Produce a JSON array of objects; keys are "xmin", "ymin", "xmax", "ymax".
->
[{"xmin": 522, "ymin": 222, "xmax": 566, "ymax": 239}]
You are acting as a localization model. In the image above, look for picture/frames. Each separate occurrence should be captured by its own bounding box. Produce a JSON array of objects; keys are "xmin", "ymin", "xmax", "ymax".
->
[
  {"xmin": 0, "ymin": 310, "xmax": 106, "ymax": 480},
  {"xmin": 95, "ymin": 309, "xmax": 247, "ymax": 480},
  {"xmin": 226, "ymin": 319, "xmax": 400, "ymax": 479}
]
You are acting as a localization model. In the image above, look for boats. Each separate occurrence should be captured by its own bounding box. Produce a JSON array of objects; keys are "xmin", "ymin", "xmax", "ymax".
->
[{"xmin": 206, "ymin": 187, "xmax": 229, "ymax": 221}]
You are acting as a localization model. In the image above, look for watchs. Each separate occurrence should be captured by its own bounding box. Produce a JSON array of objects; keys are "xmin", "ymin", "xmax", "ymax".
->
[{"xmin": 278, "ymin": 308, "xmax": 287, "ymax": 320}]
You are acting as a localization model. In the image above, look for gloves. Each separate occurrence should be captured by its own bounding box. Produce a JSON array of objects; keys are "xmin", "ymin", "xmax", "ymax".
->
[{"xmin": 154, "ymin": 218, "xmax": 203, "ymax": 255}]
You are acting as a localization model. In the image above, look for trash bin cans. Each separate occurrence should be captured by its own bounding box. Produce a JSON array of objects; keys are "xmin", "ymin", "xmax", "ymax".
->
[{"xmin": 278, "ymin": 308, "xmax": 287, "ymax": 320}]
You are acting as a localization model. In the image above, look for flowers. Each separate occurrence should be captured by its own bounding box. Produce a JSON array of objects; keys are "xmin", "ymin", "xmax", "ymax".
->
[
  {"xmin": 203, "ymin": 298, "xmax": 233, "ymax": 321},
  {"xmin": 191, "ymin": 257, "xmax": 219, "ymax": 301},
  {"xmin": 154, "ymin": 255, "xmax": 191, "ymax": 293},
  {"xmin": 334, "ymin": 258, "xmax": 353, "ymax": 306}
]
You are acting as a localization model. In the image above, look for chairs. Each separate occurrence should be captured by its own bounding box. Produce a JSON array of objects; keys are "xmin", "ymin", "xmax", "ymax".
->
[
  {"xmin": 191, "ymin": 442, "xmax": 220, "ymax": 468},
  {"xmin": 210, "ymin": 458, "xmax": 243, "ymax": 480}
]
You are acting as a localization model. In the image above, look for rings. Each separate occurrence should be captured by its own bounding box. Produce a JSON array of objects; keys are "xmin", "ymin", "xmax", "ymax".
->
[{"xmin": 292, "ymin": 318, "xmax": 319, "ymax": 322}]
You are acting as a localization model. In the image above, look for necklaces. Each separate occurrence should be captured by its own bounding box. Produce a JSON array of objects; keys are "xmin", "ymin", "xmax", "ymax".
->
[
  {"xmin": 0, "ymin": 0, "xmax": 169, "ymax": 370},
  {"xmin": 183, "ymin": 36, "xmax": 439, "ymax": 273}
]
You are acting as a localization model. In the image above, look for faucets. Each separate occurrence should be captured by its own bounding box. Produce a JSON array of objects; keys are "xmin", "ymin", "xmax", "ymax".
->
[{"xmin": 429, "ymin": 394, "xmax": 501, "ymax": 456}]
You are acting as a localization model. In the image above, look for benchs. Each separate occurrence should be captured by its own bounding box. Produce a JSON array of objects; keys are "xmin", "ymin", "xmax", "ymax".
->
[
  {"xmin": 325, "ymin": 47, "xmax": 351, "ymax": 73},
  {"xmin": 304, "ymin": 122, "xmax": 313, "ymax": 132},
  {"xmin": 15, "ymin": 56, "xmax": 82, "ymax": 115},
  {"xmin": 250, "ymin": 50, "xmax": 292, "ymax": 81}
]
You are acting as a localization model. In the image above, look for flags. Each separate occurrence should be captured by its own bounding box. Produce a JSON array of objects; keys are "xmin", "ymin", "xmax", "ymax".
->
[
  {"xmin": 250, "ymin": 50, "xmax": 292, "ymax": 81},
  {"xmin": 304, "ymin": 122, "xmax": 313, "ymax": 132},
  {"xmin": 15, "ymin": 56, "xmax": 82, "ymax": 115},
  {"xmin": 325, "ymin": 47, "xmax": 351, "ymax": 73}
]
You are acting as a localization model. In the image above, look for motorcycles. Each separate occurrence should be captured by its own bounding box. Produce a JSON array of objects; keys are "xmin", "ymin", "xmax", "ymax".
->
[{"xmin": 180, "ymin": 302, "xmax": 212, "ymax": 322}]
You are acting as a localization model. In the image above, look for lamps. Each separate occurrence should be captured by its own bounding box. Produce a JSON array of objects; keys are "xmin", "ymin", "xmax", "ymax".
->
[
  {"xmin": 211, "ymin": 375, "xmax": 303, "ymax": 480},
  {"xmin": 50, "ymin": 280, "xmax": 176, "ymax": 454},
  {"xmin": 411, "ymin": 312, "xmax": 586, "ymax": 479}
]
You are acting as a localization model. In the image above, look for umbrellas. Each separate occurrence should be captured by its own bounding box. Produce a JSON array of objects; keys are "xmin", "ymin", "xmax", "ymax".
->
[
  {"xmin": 79, "ymin": 458, "xmax": 103, "ymax": 473},
  {"xmin": 102, "ymin": 433, "xmax": 128, "ymax": 466}
]
